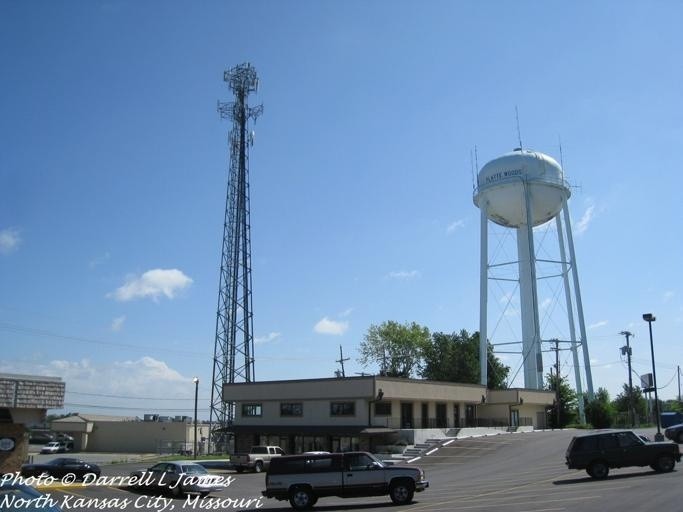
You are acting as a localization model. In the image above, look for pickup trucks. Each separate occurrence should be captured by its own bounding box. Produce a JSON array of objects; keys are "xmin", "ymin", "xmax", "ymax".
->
[
  {"xmin": 41, "ymin": 441, "xmax": 66, "ymax": 454},
  {"xmin": 261, "ymin": 451, "xmax": 428, "ymax": 511},
  {"xmin": 230, "ymin": 445, "xmax": 288, "ymax": 473}
]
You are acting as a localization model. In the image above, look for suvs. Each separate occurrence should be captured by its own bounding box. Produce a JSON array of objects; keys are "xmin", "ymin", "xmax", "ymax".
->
[{"xmin": 565, "ymin": 431, "xmax": 680, "ymax": 479}]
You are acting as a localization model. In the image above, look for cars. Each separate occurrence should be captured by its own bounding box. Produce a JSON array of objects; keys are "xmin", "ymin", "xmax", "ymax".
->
[
  {"xmin": 665, "ymin": 423, "xmax": 683, "ymax": 444},
  {"xmin": 131, "ymin": 461, "xmax": 225, "ymax": 499},
  {"xmin": 56, "ymin": 434, "xmax": 73, "ymax": 441},
  {"xmin": 20, "ymin": 458, "xmax": 100, "ymax": 483},
  {"xmin": 30, "ymin": 434, "xmax": 53, "ymax": 444}
]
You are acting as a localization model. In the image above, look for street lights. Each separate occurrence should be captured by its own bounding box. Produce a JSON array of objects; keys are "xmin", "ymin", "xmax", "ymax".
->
[
  {"xmin": 192, "ymin": 377, "xmax": 200, "ymax": 461},
  {"xmin": 642, "ymin": 312, "xmax": 660, "ymax": 432}
]
